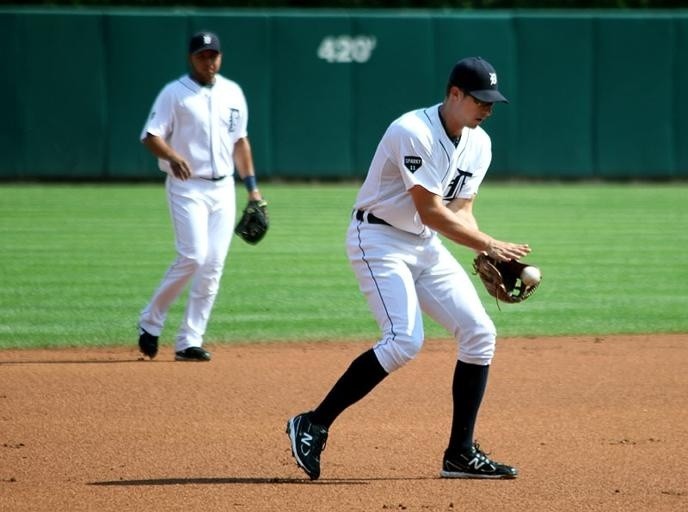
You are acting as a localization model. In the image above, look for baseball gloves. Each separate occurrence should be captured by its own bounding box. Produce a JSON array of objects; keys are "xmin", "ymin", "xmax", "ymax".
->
[
  {"xmin": 474, "ymin": 252, "xmax": 538, "ymax": 303},
  {"xmin": 235, "ymin": 197, "xmax": 269, "ymax": 246}
]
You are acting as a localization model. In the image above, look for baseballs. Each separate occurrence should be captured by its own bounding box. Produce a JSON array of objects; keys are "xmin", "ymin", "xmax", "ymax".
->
[{"xmin": 522, "ymin": 265, "xmax": 542, "ymax": 287}]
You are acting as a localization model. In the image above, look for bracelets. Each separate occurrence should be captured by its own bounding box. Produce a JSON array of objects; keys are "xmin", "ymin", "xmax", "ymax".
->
[{"xmin": 243, "ymin": 176, "xmax": 259, "ymax": 192}]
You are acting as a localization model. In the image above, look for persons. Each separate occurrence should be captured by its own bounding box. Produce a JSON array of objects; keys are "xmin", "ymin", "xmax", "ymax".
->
[
  {"xmin": 284, "ymin": 54, "xmax": 545, "ymax": 483},
  {"xmin": 136, "ymin": 30, "xmax": 270, "ymax": 362}
]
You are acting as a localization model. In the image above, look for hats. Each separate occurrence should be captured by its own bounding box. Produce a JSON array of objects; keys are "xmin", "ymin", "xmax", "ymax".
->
[
  {"xmin": 189, "ymin": 32, "xmax": 220, "ymax": 55},
  {"xmin": 450, "ymin": 56, "xmax": 510, "ymax": 104}
]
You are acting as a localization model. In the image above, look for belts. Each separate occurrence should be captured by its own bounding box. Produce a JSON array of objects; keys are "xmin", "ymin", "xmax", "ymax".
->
[
  {"xmin": 351, "ymin": 208, "xmax": 392, "ymax": 226},
  {"xmin": 200, "ymin": 176, "xmax": 225, "ymax": 181}
]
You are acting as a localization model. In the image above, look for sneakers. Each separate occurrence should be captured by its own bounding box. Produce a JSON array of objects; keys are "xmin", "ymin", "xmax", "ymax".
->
[
  {"xmin": 174, "ymin": 347, "xmax": 212, "ymax": 361},
  {"xmin": 441, "ymin": 445, "xmax": 516, "ymax": 478},
  {"xmin": 287, "ymin": 411, "xmax": 328, "ymax": 480},
  {"xmin": 138, "ymin": 327, "xmax": 159, "ymax": 358}
]
